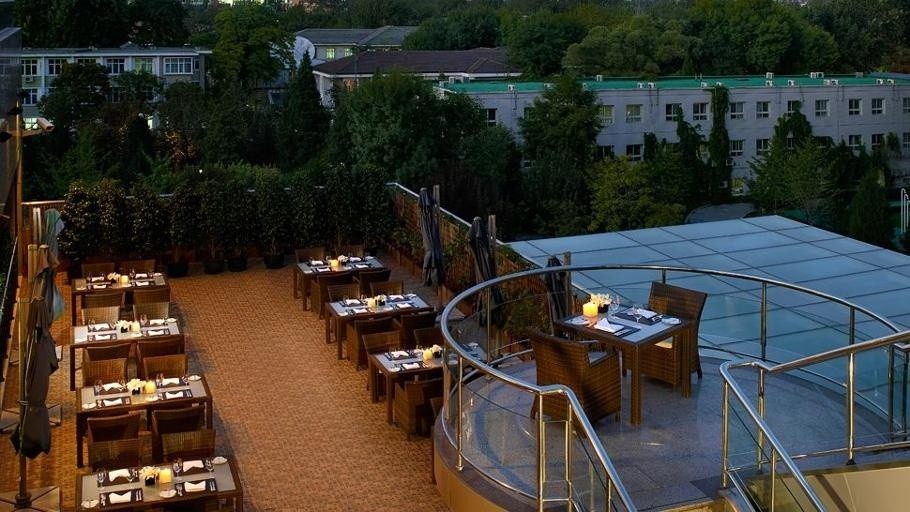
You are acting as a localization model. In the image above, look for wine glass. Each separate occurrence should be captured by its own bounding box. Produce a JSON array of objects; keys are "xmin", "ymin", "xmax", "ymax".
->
[
  {"xmin": 86, "ymin": 270, "xmax": 154, "ymax": 284},
  {"xmin": 88, "ymin": 312, "xmax": 168, "ymax": 335},
  {"xmin": 205, "ymin": 458, "xmax": 216, "ymax": 480},
  {"xmin": 94, "ymin": 468, "xmax": 109, "ymax": 490},
  {"xmin": 125, "ymin": 466, "xmax": 135, "ymax": 489},
  {"xmin": 609, "ymin": 296, "xmax": 621, "ymax": 319},
  {"xmin": 632, "ymin": 303, "xmax": 644, "ymax": 329},
  {"xmin": 343, "ymin": 290, "xmax": 414, "ymax": 303},
  {"xmin": 95, "ymin": 373, "xmax": 189, "ymax": 392},
  {"xmin": 309, "ymin": 254, "xmax": 366, "ymax": 266},
  {"xmin": 389, "ymin": 344, "xmax": 424, "ymax": 360},
  {"xmin": 173, "ymin": 458, "xmax": 184, "ymax": 481}
]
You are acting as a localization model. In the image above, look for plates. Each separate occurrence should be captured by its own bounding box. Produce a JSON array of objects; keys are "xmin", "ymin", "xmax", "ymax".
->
[
  {"xmin": 212, "ymin": 457, "xmax": 228, "ymax": 464},
  {"xmin": 662, "ymin": 317, "xmax": 681, "ymax": 325},
  {"xmin": 389, "ymin": 367, "xmax": 399, "ymax": 372},
  {"xmin": 303, "ymin": 270, "xmax": 312, "ymax": 274},
  {"xmin": 159, "ymin": 489, "xmax": 176, "ymax": 499},
  {"xmin": 154, "ymin": 272, "xmax": 162, "ymax": 276},
  {"xmin": 81, "ymin": 500, "xmax": 99, "ymax": 508},
  {"xmin": 80, "ymin": 403, "xmax": 97, "ymax": 410},
  {"xmin": 167, "ymin": 318, "xmax": 177, "ymax": 323},
  {"xmin": 74, "ymin": 338, "xmax": 88, "ymax": 342},
  {"xmin": 366, "ymin": 256, "xmax": 374, "ymax": 260},
  {"xmin": 469, "ymin": 342, "xmax": 478, "ymax": 346},
  {"xmin": 188, "ymin": 375, "xmax": 201, "ymax": 381},
  {"xmin": 77, "ymin": 287, "xmax": 86, "ymax": 291},
  {"xmin": 146, "ymin": 396, "xmax": 158, "ymax": 402}
]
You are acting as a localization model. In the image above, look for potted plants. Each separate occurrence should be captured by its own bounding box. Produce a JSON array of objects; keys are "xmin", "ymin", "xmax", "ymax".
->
[
  {"xmin": 385, "ymin": 217, "xmax": 550, "ymax": 362},
  {"xmin": 54, "ymin": 157, "xmax": 397, "ymax": 274}
]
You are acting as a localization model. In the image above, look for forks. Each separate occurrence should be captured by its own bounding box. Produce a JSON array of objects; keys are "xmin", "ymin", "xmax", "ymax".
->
[
  {"xmin": 174, "ymin": 483, "xmax": 185, "ymax": 497},
  {"xmin": 98, "ymin": 495, "xmax": 106, "ymax": 506},
  {"xmin": 97, "ymin": 399, "xmax": 103, "ymax": 408}
]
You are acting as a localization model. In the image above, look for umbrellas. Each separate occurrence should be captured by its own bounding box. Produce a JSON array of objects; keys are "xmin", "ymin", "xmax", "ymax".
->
[
  {"xmin": 34, "ymin": 244, "xmax": 61, "ymax": 279},
  {"xmin": 41, "ymin": 207, "xmax": 66, "ymax": 260},
  {"xmin": 541, "ymin": 250, "xmax": 572, "ymax": 339},
  {"xmin": 37, "ymin": 265, "xmax": 65, "ymax": 324},
  {"xmin": 416, "ymin": 181, "xmax": 450, "ymax": 314},
  {"xmin": 464, "ymin": 212, "xmax": 507, "ymax": 359},
  {"xmin": 7, "ymin": 294, "xmax": 61, "ymax": 500}
]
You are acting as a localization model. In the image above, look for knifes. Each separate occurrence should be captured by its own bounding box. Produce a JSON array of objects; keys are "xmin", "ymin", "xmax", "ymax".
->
[
  {"xmin": 398, "ymin": 363, "xmax": 405, "ymax": 371},
  {"xmin": 88, "ymin": 335, "xmax": 93, "ymax": 342},
  {"xmin": 613, "ymin": 329, "xmax": 632, "ymax": 336},
  {"xmin": 209, "ymin": 480, "xmax": 216, "ymax": 492},
  {"xmin": 311, "ymin": 268, "xmax": 315, "ymax": 273},
  {"xmin": 405, "ymin": 293, "xmax": 417, "ymax": 298},
  {"xmin": 186, "ymin": 389, "xmax": 192, "ymax": 397},
  {"xmin": 390, "ymin": 304, "xmax": 396, "ymax": 310},
  {"xmin": 125, "ymin": 397, "xmax": 130, "ymax": 405},
  {"xmin": 586, "ymin": 319, "xmax": 597, "ymax": 327},
  {"xmin": 462, "ymin": 343, "xmax": 468, "ymax": 348},
  {"xmin": 417, "ymin": 360, "xmax": 428, "ymax": 368},
  {"xmin": 652, "ymin": 315, "xmax": 664, "ymax": 322},
  {"xmin": 135, "ymin": 489, "xmax": 143, "ymax": 501}
]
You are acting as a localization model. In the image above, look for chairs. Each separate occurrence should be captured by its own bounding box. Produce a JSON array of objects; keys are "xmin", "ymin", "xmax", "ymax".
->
[
  {"xmin": 621, "ymin": 276, "xmax": 709, "ymax": 401},
  {"xmin": 525, "ymin": 329, "xmax": 623, "ymax": 439},
  {"xmin": 292, "ymin": 235, "xmax": 493, "ymax": 440},
  {"xmin": 67, "ymin": 253, "xmax": 243, "ymax": 512}
]
